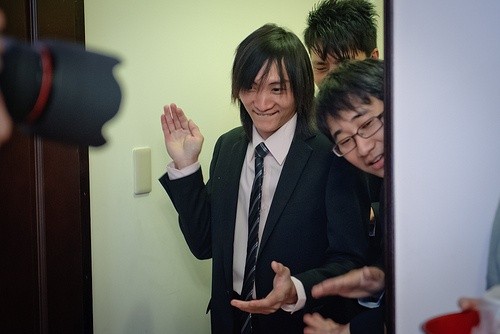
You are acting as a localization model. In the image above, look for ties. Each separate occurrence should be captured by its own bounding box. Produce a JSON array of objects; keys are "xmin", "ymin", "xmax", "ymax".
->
[{"xmin": 239, "ymin": 142, "xmax": 269, "ymax": 334}]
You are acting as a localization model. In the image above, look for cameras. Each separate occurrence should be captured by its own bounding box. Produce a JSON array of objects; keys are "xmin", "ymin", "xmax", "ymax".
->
[{"xmin": 0, "ymin": 40, "xmax": 122, "ymax": 147}]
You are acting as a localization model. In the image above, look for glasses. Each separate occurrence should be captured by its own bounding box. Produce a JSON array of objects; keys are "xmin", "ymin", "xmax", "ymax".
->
[{"xmin": 332, "ymin": 111, "xmax": 384, "ymax": 156}]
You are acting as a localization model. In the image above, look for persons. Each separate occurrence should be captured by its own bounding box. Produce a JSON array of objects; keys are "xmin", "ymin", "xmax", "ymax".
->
[
  {"xmin": 458, "ymin": 200, "xmax": 500, "ymax": 334},
  {"xmin": 303, "ymin": 0, "xmax": 381, "ymax": 93},
  {"xmin": 304, "ymin": 59, "xmax": 396, "ymax": 334},
  {"xmin": 160, "ymin": 23, "xmax": 373, "ymax": 334}
]
